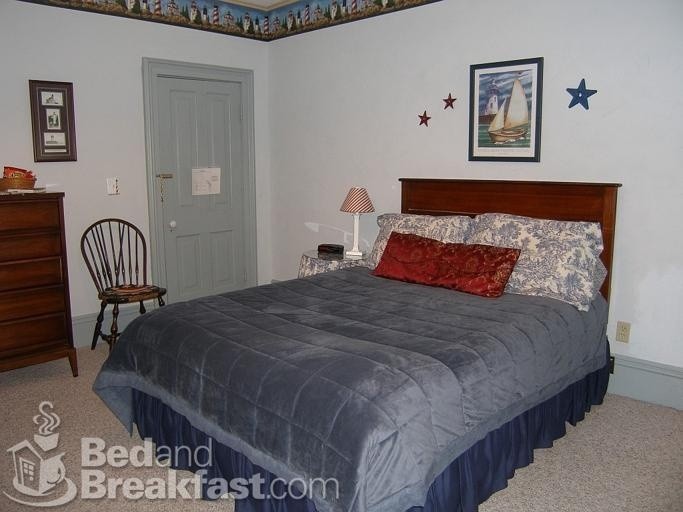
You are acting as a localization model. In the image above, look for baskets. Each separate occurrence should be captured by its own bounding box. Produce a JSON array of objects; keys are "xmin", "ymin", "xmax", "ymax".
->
[{"xmin": 0, "ymin": 177, "xmax": 36, "ymax": 189}]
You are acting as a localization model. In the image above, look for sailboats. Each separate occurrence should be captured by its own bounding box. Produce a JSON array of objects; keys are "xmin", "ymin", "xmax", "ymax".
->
[{"xmin": 479, "ymin": 80, "xmax": 528, "ymax": 142}]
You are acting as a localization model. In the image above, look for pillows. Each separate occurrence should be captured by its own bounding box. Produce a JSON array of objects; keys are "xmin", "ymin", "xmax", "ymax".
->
[{"xmin": 365, "ymin": 211, "xmax": 608, "ymax": 313}]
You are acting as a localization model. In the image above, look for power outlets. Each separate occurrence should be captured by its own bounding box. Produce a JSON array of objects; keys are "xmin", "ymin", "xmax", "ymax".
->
[{"xmin": 616, "ymin": 321, "xmax": 631, "ymax": 344}]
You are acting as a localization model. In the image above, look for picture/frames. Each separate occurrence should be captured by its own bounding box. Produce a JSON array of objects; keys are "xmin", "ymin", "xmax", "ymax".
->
[
  {"xmin": 28, "ymin": 79, "xmax": 78, "ymax": 162},
  {"xmin": 467, "ymin": 55, "xmax": 545, "ymax": 162}
]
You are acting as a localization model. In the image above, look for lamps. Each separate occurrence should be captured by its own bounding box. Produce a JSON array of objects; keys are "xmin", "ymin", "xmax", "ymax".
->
[{"xmin": 340, "ymin": 186, "xmax": 376, "ymax": 257}]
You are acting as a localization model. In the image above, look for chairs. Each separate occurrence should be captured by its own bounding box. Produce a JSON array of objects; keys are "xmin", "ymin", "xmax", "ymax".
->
[{"xmin": 80, "ymin": 218, "xmax": 167, "ymax": 354}]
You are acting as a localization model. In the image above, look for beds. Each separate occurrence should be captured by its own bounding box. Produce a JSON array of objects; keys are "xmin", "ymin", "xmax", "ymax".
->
[{"xmin": 90, "ymin": 177, "xmax": 623, "ymax": 512}]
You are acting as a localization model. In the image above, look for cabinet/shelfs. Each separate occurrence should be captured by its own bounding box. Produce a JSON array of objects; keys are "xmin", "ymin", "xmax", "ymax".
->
[{"xmin": 0, "ymin": 188, "xmax": 78, "ymax": 379}]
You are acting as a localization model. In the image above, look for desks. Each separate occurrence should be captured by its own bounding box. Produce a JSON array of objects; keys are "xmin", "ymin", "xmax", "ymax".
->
[{"xmin": 298, "ymin": 249, "xmax": 369, "ymax": 278}]
